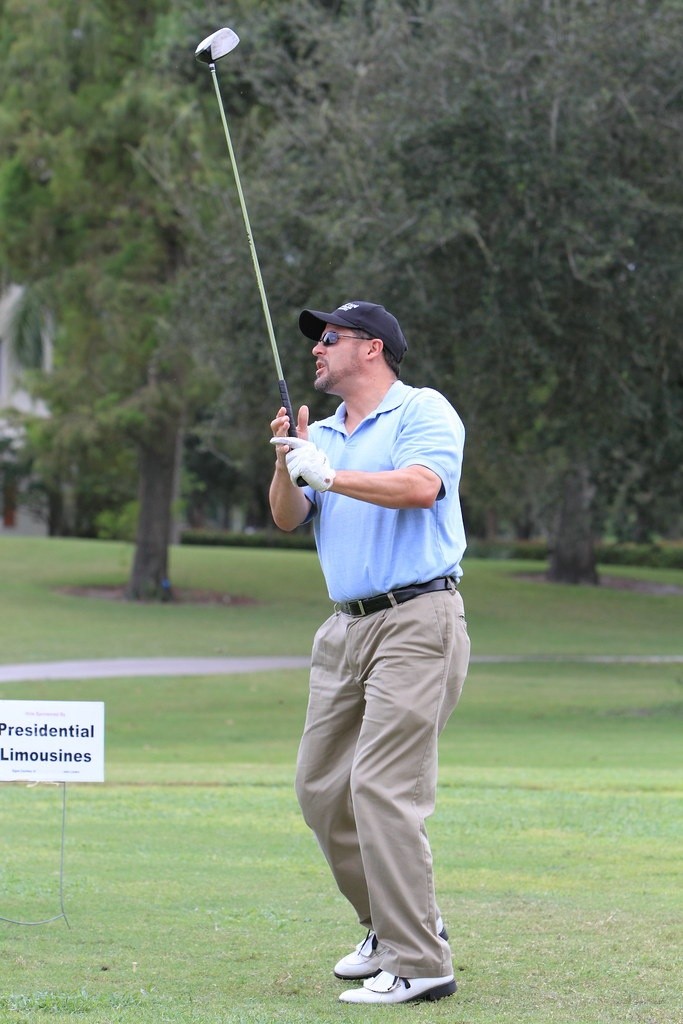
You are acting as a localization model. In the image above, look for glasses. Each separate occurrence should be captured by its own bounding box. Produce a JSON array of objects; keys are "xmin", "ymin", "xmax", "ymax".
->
[{"xmin": 318, "ymin": 331, "xmax": 373, "ymax": 346}]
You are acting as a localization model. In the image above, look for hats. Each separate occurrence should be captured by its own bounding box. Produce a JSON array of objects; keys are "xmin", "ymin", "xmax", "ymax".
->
[{"xmin": 299, "ymin": 301, "xmax": 408, "ymax": 364}]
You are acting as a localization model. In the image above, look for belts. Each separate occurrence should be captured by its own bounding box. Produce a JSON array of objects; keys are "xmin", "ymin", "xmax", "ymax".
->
[{"xmin": 341, "ymin": 576, "xmax": 457, "ymax": 616}]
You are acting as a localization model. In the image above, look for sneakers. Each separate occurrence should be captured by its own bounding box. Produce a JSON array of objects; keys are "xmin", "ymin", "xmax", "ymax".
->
[
  {"xmin": 339, "ymin": 968, "xmax": 457, "ymax": 1003},
  {"xmin": 334, "ymin": 915, "xmax": 449, "ymax": 979}
]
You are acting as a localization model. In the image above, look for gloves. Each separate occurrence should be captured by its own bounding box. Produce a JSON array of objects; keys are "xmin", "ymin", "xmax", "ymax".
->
[{"xmin": 270, "ymin": 437, "xmax": 336, "ymax": 493}]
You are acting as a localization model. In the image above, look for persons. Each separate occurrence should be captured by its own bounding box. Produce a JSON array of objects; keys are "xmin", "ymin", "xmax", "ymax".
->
[{"xmin": 268, "ymin": 299, "xmax": 472, "ymax": 1006}]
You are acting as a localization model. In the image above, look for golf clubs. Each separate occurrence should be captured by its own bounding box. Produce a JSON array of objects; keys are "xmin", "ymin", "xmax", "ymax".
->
[{"xmin": 194, "ymin": 27, "xmax": 309, "ymax": 489}]
